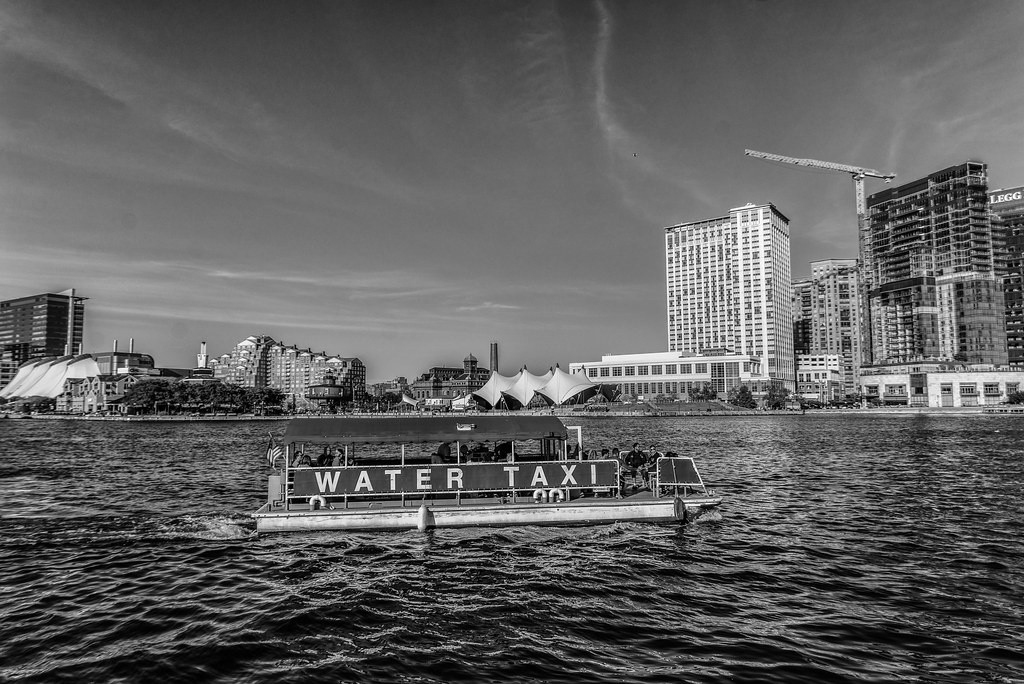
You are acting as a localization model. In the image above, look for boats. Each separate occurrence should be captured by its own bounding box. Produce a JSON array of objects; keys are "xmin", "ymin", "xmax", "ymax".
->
[{"xmin": 248, "ymin": 415, "xmax": 720, "ymax": 533}]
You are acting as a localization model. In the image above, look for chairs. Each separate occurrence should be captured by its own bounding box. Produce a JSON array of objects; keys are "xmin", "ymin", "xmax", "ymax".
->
[{"xmin": 618, "ymin": 450, "xmax": 710, "ymax": 499}]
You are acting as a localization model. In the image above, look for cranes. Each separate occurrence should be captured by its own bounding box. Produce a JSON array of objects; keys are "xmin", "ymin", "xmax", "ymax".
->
[{"xmin": 744, "ymin": 148, "xmax": 897, "ymax": 367}]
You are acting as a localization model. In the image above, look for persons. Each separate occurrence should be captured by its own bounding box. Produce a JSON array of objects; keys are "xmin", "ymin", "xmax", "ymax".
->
[{"xmin": 290, "ymin": 441, "xmax": 681, "ymax": 492}]
